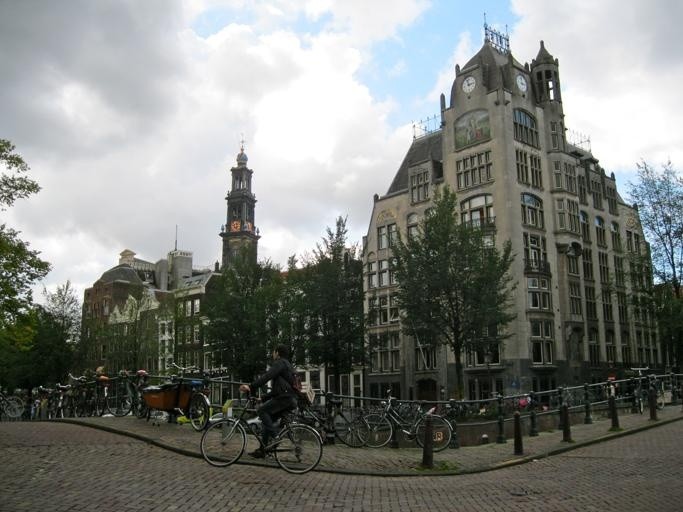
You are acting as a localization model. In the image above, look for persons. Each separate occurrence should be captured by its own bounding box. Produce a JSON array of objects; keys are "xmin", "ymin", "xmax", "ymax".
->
[{"xmin": 239, "ymin": 343, "xmax": 297, "ymax": 458}]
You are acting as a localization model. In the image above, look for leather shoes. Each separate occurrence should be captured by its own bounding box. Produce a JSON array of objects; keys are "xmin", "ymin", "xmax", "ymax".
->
[{"xmin": 248, "ymin": 438, "xmax": 281, "ymax": 459}]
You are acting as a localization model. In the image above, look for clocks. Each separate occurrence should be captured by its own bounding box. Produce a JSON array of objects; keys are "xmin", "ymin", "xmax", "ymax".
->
[
  {"xmin": 514, "ymin": 73, "xmax": 528, "ymax": 92},
  {"xmin": 460, "ymin": 75, "xmax": 476, "ymax": 94}
]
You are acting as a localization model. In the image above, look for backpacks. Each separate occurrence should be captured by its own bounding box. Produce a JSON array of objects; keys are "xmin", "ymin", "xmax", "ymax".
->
[{"xmin": 280, "ymin": 359, "xmax": 302, "ymax": 394}]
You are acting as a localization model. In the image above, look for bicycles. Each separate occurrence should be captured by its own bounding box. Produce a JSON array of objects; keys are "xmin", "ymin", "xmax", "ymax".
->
[
  {"xmin": 0, "ymin": 362, "xmax": 211, "ymax": 431},
  {"xmin": 630, "ymin": 366, "xmax": 665, "ymax": 415},
  {"xmin": 200, "ymin": 385, "xmax": 454, "ymax": 475}
]
